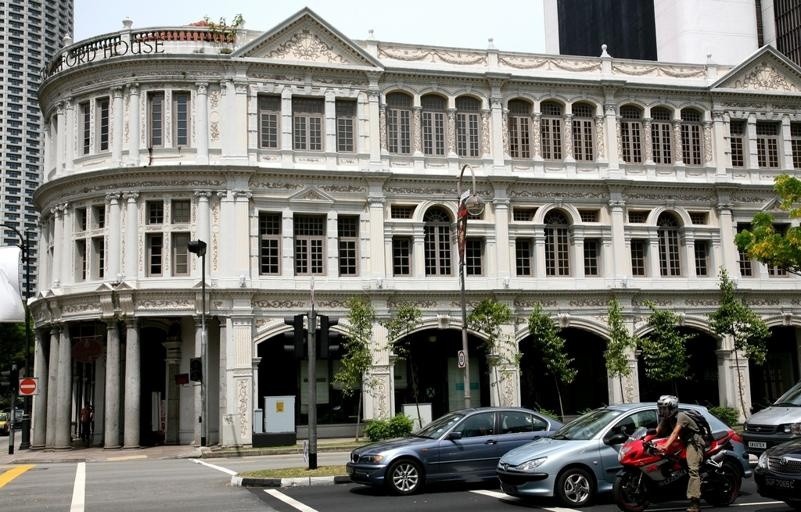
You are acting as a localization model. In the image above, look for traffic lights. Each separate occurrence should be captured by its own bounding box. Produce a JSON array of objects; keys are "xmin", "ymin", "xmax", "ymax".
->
[
  {"xmin": 189, "ymin": 356, "xmax": 202, "ymax": 383},
  {"xmin": 0, "ymin": 362, "xmax": 15, "ymax": 395},
  {"xmin": 283, "ymin": 315, "xmax": 304, "ymax": 358},
  {"xmin": 320, "ymin": 315, "xmax": 340, "ymax": 358},
  {"xmin": 185, "ymin": 238, "xmax": 207, "ymax": 259}
]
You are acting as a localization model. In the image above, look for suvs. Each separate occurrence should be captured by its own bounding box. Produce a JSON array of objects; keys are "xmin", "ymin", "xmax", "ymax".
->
[{"xmin": 739, "ymin": 379, "xmax": 801, "ymax": 460}]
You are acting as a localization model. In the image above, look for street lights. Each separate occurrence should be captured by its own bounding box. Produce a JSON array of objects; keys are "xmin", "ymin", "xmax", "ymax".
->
[{"xmin": 453, "ymin": 161, "xmax": 485, "ymax": 409}]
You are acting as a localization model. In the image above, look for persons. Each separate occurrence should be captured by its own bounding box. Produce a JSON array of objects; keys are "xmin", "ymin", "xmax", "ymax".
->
[
  {"xmin": 656, "ymin": 395, "xmax": 706, "ymax": 512},
  {"xmin": 80, "ymin": 402, "xmax": 94, "ymax": 446}
]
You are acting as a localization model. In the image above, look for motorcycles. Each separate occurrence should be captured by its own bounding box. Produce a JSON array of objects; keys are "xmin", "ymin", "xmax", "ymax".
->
[{"xmin": 610, "ymin": 426, "xmax": 746, "ymax": 511}]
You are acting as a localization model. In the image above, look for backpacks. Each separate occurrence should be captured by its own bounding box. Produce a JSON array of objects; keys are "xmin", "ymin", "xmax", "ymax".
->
[{"xmin": 672, "ymin": 408, "xmax": 715, "ymax": 449}]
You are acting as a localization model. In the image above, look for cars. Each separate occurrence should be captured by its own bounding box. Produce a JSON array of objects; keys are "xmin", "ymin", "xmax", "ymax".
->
[
  {"xmin": 343, "ymin": 405, "xmax": 571, "ymax": 497},
  {"xmin": 0, "ymin": 406, "xmax": 29, "ymax": 438},
  {"xmin": 494, "ymin": 398, "xmax": 754, "ymax": 509},
  {"xmin": 753, "ymin": 437, "xmax": 801, "ymax": 512}
]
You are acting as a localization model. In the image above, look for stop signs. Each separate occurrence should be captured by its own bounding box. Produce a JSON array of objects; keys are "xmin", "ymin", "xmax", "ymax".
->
[{"xmin": 19, "ymin": 378, "xmax": 38, "ymax": 395}]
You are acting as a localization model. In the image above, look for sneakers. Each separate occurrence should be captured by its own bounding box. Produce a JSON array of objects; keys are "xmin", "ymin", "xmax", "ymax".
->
[{"xmin": 686, "ymin": 504, "xmax": 700, "ymax": 512}]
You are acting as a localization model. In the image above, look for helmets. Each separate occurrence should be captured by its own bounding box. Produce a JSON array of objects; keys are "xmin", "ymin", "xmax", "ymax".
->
[{"xmin": 657, "ymin": 394, "xmax": 679, "ymax": 407}]
